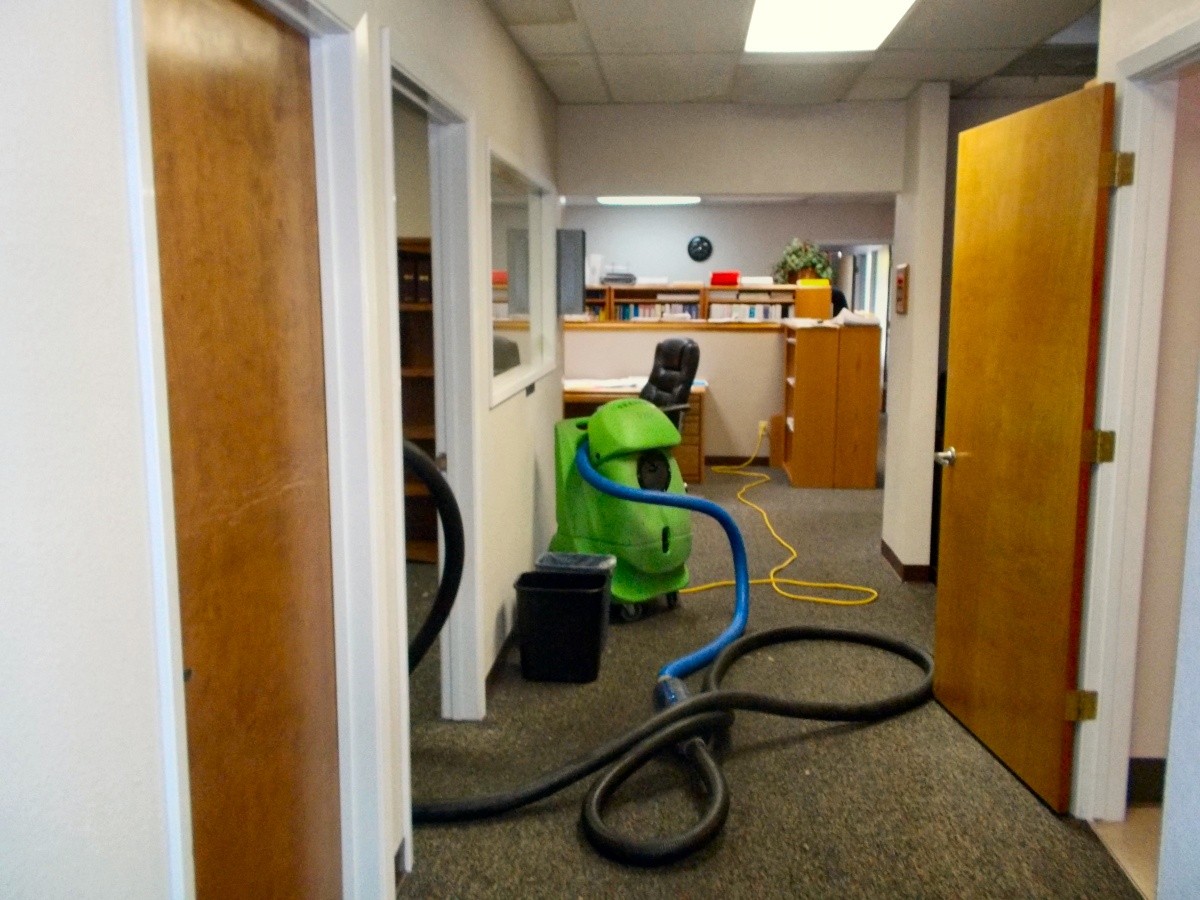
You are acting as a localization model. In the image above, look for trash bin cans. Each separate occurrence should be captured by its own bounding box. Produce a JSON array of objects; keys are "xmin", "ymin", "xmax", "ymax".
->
[
  {"xmin": 513, "ymin": 571, "xmax": 609, "ymax": 684},
  {"xmin": 535, "ymin": 552, "xmax": 616, "ymax": 572}
]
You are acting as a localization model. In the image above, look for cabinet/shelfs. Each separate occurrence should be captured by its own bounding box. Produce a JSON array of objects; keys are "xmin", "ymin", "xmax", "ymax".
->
[
  {"xmin": 834, "ymin": 325, "xmax": 885, "ymax": 487},
  {"xmin": 704, "ymin": 283, "xmax": 797, "ymax": 320},
  {"xmin": 610, "ymin": 283, "xmax": 705, "ymax": 321},
  {"xmin": 781, "ymin": 322, "xmax": 840, "ymax": 488},
  {"xmin": 585, "ymin": 285, "xmax": 611, "ymax": 319},
  {"xmin": 397, "ymin": 237, "xmax": 438, "ymax": 558}
]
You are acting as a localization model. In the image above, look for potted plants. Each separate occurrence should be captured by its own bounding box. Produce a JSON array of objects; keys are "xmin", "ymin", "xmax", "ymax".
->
[{"xmin": 772, "ymin": 236, "xmax": 836, "ymax": 284}]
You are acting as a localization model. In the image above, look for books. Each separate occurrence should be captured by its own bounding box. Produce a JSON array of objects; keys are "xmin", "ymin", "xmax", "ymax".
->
[{"xmin": 564, "ymin": 273, "xmax": 880, "ymax": 330}]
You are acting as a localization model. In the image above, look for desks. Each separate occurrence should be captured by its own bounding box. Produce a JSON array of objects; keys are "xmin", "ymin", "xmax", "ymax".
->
[{"xmin": 562, "ymin": 386, "xmax": 707, "ymax": 484}]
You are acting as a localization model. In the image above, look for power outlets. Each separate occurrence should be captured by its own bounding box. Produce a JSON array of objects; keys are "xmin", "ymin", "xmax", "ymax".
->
[{"xmin": 757, "ymin": 419, "xmax": 770, "ymax": 437}]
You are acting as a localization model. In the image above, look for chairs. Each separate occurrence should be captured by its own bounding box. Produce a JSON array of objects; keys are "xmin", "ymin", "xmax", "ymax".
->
[{"xmin": 640, "ymin": 336, "xmax": 699, "ymax": 431}]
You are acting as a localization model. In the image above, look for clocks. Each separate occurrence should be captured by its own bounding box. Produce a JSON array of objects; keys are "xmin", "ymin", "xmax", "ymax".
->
[{"xmin": 688, "ymin": 236, "xmax": 712, "ymax": 262}]
